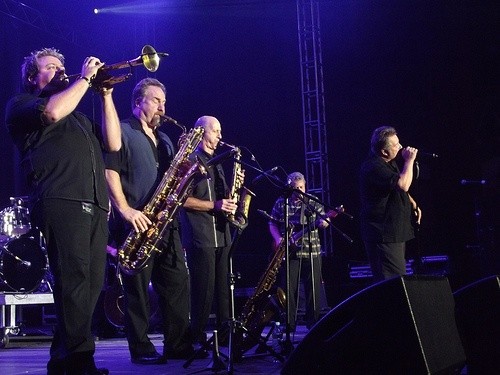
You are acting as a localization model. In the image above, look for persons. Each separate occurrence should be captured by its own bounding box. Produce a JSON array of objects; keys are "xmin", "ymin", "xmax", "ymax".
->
[
  {"xmin": 175, "ymin": 116, "xmax": 246, "ymax": 349},
  {"xmin": 104, "ymin": 76, "xmax": 209, "ymax": 365},
  {"xmin": 269, "ymin": 171, "xmax": 330, "ymax": 332},
  {"xmin": 359, "ymin": 127, "xmax": 422, "ymax": 281},
  {"xmin": 6, "ymin": 48, "xmax": 122, "ymax": 375}
]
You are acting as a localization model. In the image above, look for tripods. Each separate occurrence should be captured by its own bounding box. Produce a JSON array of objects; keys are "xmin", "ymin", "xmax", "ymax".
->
[{"xmin": 182, "ymin": 227, "xmax": 286, "ymax": 375}]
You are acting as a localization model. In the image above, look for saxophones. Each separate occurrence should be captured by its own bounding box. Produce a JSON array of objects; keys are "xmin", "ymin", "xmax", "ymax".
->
[
  {"xmin": 225, "ymin": 207, "xmax": 293, "ymax": 348},
  {"xmin": 117, "ymin": 112, "xmax": 208, "ymax": 276},
  {"xmin": 218, "ymin": 140, "xmax": 257, "ymax": 229}
]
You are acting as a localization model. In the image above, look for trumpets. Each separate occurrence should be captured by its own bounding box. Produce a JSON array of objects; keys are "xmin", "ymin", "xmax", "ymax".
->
[{"xmin": 59, "ymin": 43, "xmax": 160, "ymax": 91}]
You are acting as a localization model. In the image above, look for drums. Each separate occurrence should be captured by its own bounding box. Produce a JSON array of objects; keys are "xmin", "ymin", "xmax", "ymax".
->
[{"xmin": 0, "ymin": 196, "xmax": 52, "ymax": 294}]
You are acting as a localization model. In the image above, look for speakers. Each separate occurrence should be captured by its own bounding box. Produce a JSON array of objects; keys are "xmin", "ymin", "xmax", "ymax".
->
[{"xmin": 279, "ymin": 272, "xmax": 500, "ymax": 375}]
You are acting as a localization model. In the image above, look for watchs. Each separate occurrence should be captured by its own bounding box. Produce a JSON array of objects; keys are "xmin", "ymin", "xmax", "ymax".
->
[{"xmin": 77, "ymin": 75, "xmax": 91, "ymax": 86}]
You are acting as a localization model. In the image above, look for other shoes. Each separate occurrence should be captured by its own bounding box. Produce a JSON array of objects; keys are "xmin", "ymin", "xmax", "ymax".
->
[
  {"xmin": 95, "ymin": 368, "xmax": 110, "ymax": 375},
  {"xmin": 163, "ymin": 345, "xmax": 206, "ymax": 360},
  {"xmin": 130, "ymin": 351, "xmax": 167, "ymax": 364}
]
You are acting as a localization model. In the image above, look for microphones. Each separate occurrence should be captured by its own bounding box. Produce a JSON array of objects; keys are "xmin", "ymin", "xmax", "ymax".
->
[
  {"xmin": 140, "ymin": 52, "xmax": 169, "ymax": 56},
  {"xmin": 206, "ymin": 147, "xmax": 239, "ymax": 166},
  {"xmin": 24, "ymin": 261, "xmax": 31, "ymax": 266},
  {"xmin": 461, "ymin": 180, "xmax": 487, "ymax": 184},
  {"xmin": 416, "ymin": 152, "xmax": 438, "ymax": 158},
  {"xmin": 252, "ymin": 167, "xmax": 279, "ymax": 185}
]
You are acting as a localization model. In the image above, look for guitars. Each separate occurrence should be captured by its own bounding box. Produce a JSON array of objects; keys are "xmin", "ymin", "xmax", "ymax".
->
[{"xmin": 272, "ymin": 204, "xmax": 346, "ymax": 260}]
